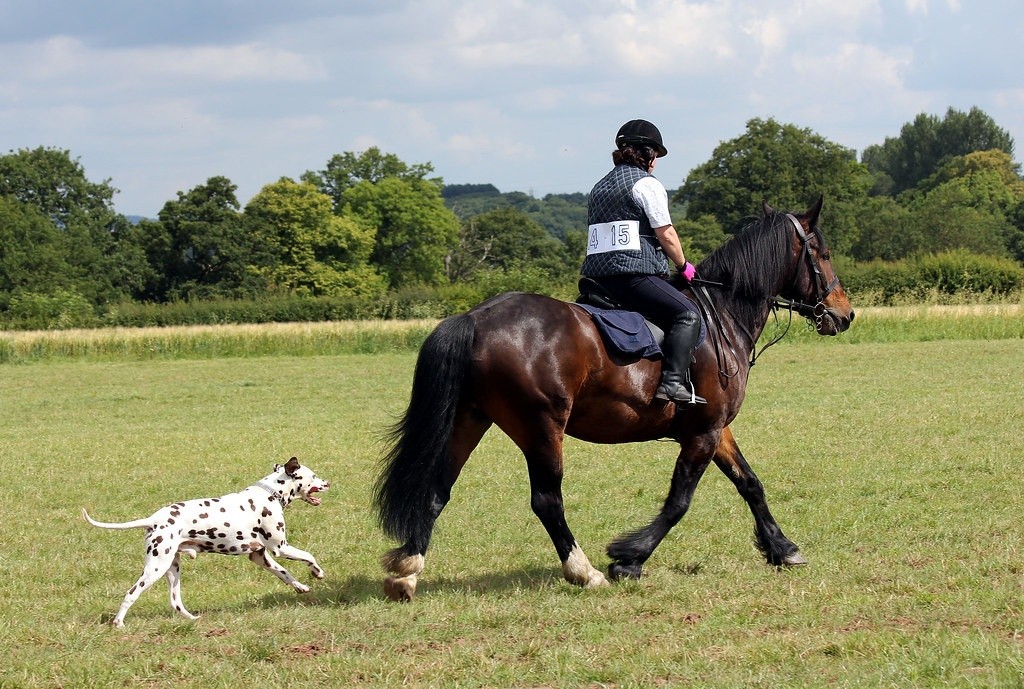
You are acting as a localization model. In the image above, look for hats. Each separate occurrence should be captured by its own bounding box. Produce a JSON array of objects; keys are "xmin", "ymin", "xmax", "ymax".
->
[{"xmin": 615, "ymin": 119, "xmax": 668, "ymax": 158}]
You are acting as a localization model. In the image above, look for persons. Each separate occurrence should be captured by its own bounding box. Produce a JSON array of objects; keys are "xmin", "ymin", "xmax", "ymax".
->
[{"xmin": 581, "ymin": 118, "xmax": 708, "ymax": 405}]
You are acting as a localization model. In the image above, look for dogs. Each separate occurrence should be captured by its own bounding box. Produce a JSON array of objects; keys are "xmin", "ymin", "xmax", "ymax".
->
[{"xmin": 80, "ymin": 457, "xmax": 333, "ymax": 628}]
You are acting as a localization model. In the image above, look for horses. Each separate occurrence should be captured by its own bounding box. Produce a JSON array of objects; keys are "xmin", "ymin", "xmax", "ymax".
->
[{"xmin": 366, "ymin": 192, "xmax": 855, "ymax": 601}]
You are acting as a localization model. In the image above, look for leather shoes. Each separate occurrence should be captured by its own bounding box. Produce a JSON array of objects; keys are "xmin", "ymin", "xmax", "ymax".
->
[{"xmin": 655, "ymin": 383, "xmax": 707, "ymax": 405}]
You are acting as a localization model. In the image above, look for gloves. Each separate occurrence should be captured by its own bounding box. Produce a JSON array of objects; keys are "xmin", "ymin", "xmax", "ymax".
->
[{"xmin": 675, "ymin": 260, "xmax": 696, "ymax": 282}]
